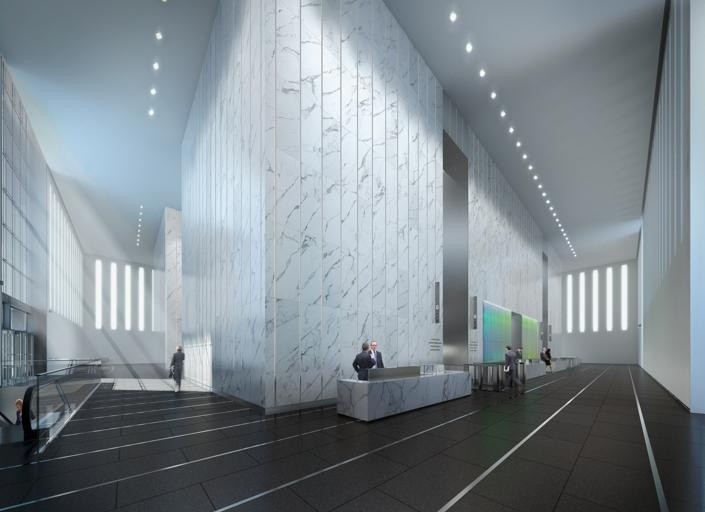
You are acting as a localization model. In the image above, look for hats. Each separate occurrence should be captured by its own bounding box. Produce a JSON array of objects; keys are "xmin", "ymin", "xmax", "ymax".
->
[{"xmin": 176, "ymin": 346, "xmax": 182, "ymax": 351}]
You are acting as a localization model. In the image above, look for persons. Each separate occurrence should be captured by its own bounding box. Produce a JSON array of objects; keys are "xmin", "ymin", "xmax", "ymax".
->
[
  {"xmin": 501, "ymin": 346, "xmax": 523, "ymax": 392},
  {"xmin": 540, "ymin": 347, "xmax": 546, "ymax": 361},
  {"xmin": 15, "ymin": 399, "xmax": 34, "ymax": 425},
  {"xmin": 368, "ymin": 340, "xmax": 384, "ymax": 368},
  {"xmin": 545, "ymin": 348, "xmax": 554, "ymax": 375},
  {"xmin": 170, "ymin": 345, "xmax": 185, "ymax": 393},
  {"xmin": 353, "ymin": 343, "xmax": 375, "ymax": 380},
  {"xmin": 515, "ymin": 347, "xmax": 522, "ymax": 358}
]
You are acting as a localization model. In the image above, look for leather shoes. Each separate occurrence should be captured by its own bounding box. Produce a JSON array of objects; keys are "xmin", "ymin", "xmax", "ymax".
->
[{"xmin": 502, "ymin": 388, "xmax": 519, "ymax": 392}]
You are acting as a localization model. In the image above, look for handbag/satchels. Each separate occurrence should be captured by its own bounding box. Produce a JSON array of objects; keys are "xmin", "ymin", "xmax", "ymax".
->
[{"xmin": 169, "ymin": 369, "xmax": 172, "ymax": 378}]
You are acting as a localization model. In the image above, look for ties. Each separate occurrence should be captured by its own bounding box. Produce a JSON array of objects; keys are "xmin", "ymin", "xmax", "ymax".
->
[{"xmin": 374, "ymin": 351, "xmax": 376, "ymax": 362}]
